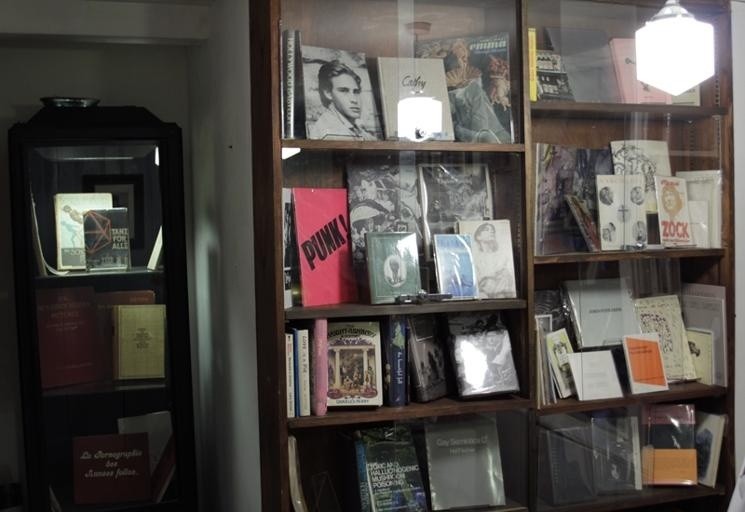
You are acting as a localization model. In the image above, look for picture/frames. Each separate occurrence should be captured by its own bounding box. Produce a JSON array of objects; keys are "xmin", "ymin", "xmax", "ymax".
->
[{"xmin": 80, "ymin": 173, "xmax": 146, "ymax": 251}]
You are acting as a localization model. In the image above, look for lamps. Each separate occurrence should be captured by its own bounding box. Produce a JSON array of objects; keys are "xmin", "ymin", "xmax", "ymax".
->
[{"xmin": 631, "ymin": 0, "xmax": 719, "ymax": 100}]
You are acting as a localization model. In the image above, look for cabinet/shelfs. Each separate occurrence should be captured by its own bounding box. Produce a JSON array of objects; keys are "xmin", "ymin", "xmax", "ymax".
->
[
  {"xmin": 7, "ymin": 101, "xmax": 199, "ymax": 509},
  {"xmin": 248, "ymin": 0, "xmax": 736, "ymax": 512}
]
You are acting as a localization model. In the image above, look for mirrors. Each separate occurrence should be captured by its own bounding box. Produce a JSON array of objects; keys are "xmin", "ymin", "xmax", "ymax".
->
[{"xmin": 22, "ymin": 131, "xmax": 174, "ymax": 277}]
